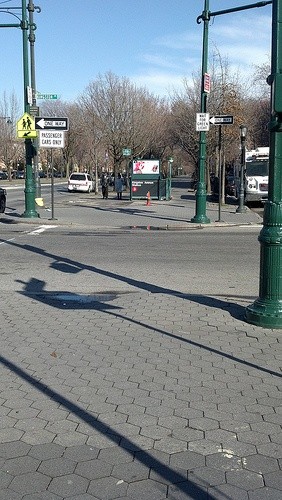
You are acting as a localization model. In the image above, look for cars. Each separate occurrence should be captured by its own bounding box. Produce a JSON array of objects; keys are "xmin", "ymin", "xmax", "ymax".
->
[
  {"xmin": 100, "ymin": 171, "xmax": 124, "ymax": 186},
  {"xmin": 0, "ymin": 168, "xmax": 66, "ymax": 180},
  {"xmin": 0, "ymin": 187, "xmax": 7, "ymax": 213},
  {"xmin": 68, "ymin": 172, "xmax": 95, "ymax": 193},
  {"xmin": 208, "ymin": 171, "xmax": 235, "ymax": 196}
]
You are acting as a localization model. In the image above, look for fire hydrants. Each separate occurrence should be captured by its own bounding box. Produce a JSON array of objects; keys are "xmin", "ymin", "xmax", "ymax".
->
[{"xmin": 145, "ymin": 191, "xmax": 150, "ymax": 206}]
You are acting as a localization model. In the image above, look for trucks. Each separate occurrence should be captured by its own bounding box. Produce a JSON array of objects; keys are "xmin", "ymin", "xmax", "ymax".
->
[{"xmin": 233, "ymin": 146, "xmax": 271, "ymax": 205}]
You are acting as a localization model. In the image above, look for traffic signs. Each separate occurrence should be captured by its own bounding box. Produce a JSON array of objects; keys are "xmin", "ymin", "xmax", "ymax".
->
[
  {"xmin": 209, "ymin": 115, "xmax": 234, "ymax": 125},
  {"xmin": 34, "ymin": 117, "xmax": 70, "ymax": 132}
]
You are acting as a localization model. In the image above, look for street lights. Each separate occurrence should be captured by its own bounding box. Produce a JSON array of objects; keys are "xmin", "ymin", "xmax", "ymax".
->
[{"xmin": 235, "ymin": 124, "xmax": 248, "ymax": 213}]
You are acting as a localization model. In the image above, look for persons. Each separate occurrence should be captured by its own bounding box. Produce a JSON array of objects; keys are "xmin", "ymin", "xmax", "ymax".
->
[
  {"xmin": 115, "ymin": 174, "xmax": 124, "ymax": 199},
  {"xmin": 100, "ymin": 174, "xmax": 110, "ymax": 200}
]
